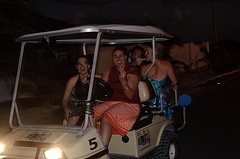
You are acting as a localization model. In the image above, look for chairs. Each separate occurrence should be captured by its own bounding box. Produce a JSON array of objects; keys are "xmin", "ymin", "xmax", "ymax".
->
[{"xmin": 133, "ymin": 80, "xmax": 157, "ymax": 130}]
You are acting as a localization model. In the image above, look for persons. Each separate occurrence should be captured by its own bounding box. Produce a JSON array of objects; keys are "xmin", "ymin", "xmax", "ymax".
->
[{"xmin": 63, "ymin": 43, "xmax": 177, "ymax": 159}]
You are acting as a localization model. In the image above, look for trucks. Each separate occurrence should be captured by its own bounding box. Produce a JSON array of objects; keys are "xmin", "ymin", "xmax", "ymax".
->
[{"xmin": 0, "ymin": 23, "xmax": 212, "ymax": 159}]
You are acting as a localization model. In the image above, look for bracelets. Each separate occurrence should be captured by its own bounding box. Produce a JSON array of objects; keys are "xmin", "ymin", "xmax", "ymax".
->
[{"xmin": 122, "ymin": 84, "xmax": 128, "ymax": 86}]
